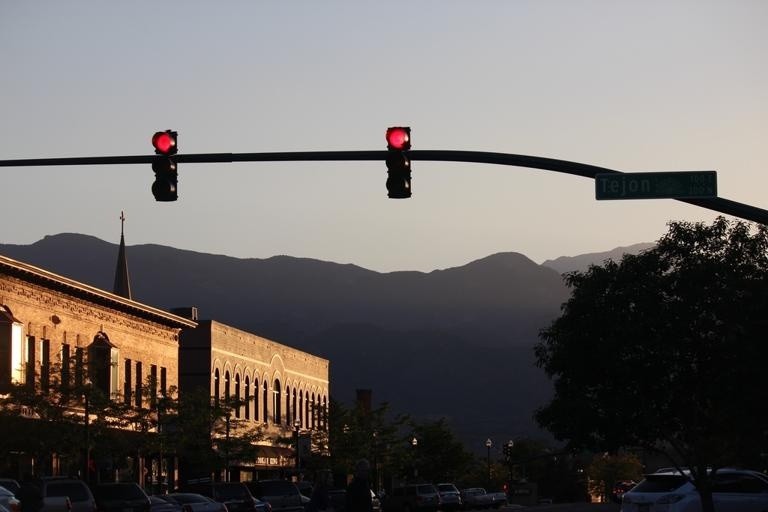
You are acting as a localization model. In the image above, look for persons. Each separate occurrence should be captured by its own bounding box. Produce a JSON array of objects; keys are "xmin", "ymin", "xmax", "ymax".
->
[
  {"xmin": 340, "ymin": 459, "xmax": 374, "ymax": 512},
  {"xmin": 304, "ymin": 469, "xmax": 336, "ymax": 512}
]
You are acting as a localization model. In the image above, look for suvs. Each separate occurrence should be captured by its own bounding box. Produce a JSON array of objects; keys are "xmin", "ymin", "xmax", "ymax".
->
[
  {"xmin": 41, "ymin": 476, "xmax": 97, "ymax": 511},
  {"xmin": 617, "ymin": 464, "xmax": 768, "ymax": 512},
  {"xmin": 178, "ymin": 479, "xmax": 255, "ymax": 512},
  {"xmin": 437, "ymin": 484, "xmax": 463, "ymax": 507},
  {"xmin": 245, "ymin": 478, "xmax": 306, "ymax": 511},
  {"xmin": 398, "ymin": 483, "xmax": 442, "ymax": 512}
]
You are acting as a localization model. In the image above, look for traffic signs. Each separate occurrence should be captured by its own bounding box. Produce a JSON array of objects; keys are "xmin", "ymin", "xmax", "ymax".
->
[{"xmin": 592, "ymin": 170, "xmax": 719, "ymax": 200}]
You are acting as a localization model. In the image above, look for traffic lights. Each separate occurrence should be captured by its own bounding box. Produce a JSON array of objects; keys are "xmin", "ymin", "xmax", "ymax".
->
[
  {"xmin": 380, "ymin": 126, "xmax": 416, "ymax": 204},
  {"xmin": 147, "ymin": 128, "xmax": 181, "ymax": 204}
]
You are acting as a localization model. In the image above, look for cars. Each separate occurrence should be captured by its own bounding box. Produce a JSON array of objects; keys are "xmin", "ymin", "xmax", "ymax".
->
[
  {"xmin": 608, "ymin": 479, "xmax": 637, "ymax": 502},
  {"xmin": 0, "ymin": 479, "xmax": 23, "ymax": 512},
  {"xmin": 93, "ymin": 481, "xmax": 152, "ymax": 512},
  {"xmin": 320, "ymin": 487, "xmax": 384, "ymax": 512},
  {"xmin": 147, "ymin": 492, "xmax": 229, "ymax": 512},
  {"xmin": 460, "ymin": 487, "xmax": 506, "ymax": 508}
]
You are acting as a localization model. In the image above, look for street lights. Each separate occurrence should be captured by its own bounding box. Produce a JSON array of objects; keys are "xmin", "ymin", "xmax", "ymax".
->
[
  {"xmin": 156, "ymin": 393, "xmax": 164, "ymax": 488},
  {"xmin": 81, "ymin": 378, "xmax": 94, "ymax": 478},
  {"xmin": 484, "ymin": 436, "xmax": 494, "ymax": 491},
  {"xmin": 410, "ymin": 436, "xmax": 420, "ymax": 446},
  {"xmin": 502, "ymin": 437, "xmax": 515, "ymax": 499},
  {"xmin": 293, "ymin": 416, "xmax": 302, "ymax": 472},
  {"xmin": 224, "ymin": 412, "xmax": 231, "ymax": 482}
]
